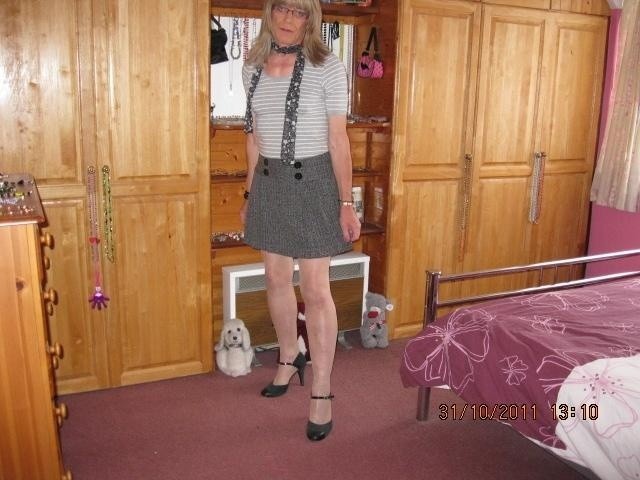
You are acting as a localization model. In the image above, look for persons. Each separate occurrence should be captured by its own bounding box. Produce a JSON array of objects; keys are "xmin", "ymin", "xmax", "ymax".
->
[{"xmin": 240, "ymin": 0, "xmax": 363, "ymax": 437}]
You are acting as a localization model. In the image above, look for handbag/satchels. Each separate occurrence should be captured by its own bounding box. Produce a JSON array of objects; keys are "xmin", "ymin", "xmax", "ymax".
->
[
  {"xmin": 210, "ymin": 28, "xmax": 229, "ymax": 64},
  {"xmin": 356, "ymin": 51, "xmax": 384, "ymax": 80}
]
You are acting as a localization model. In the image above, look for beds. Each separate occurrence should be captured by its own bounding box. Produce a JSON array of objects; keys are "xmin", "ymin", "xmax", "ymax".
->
[{"xmin": 400, "ymin": 241, "xmax": 640, "ymax": 480}]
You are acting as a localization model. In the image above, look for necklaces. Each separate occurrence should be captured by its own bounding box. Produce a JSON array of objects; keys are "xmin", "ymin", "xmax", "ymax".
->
[
  {"xmin": 101, "ymin": 165, "xmax": 115, "ymax": 261},
  {"xmin": 85, "ymin": 167, "xmax": 111, "ymax": 311},
  {"xmin": 228, "ymin": 16, "xmax": 354, "ymax": 78}
]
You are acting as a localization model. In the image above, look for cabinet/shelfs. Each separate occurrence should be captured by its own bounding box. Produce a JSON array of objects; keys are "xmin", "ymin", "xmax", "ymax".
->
[
  {"xmin": 0, "ymin": 0, "xmax": 215, "ymax": 397},
  {"xmin": 357, "ymin": 2, "xmax": 482, "ymax": 340},
  {"xmin": 465, "ymin": 2, "xmax": 613, "ymax": 299},
  {"xmin": 213, "ymin": 0, "xmax": 399, "ymax": 353},
  {"xmin": 0, "ymin": 178, "xmax": 73, "ymax": 480}
]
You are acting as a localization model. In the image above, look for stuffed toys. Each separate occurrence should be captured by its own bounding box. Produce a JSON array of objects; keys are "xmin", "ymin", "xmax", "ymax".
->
[
  {"xmin": 360, "ymin": 290, "xmax": 391, "ymax": 349},
  {"xmin": 271, "ymin": 302, "xmax": 312, "ymax": 366},
  {"xmin": 213, "ymin": 317, "xmax": 253, "ymax": 377}
]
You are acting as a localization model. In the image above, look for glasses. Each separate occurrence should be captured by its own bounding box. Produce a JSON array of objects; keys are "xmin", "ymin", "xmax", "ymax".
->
[{"xmin": 275, "ymin": 6, "xmax": 307, "ymax": 18}]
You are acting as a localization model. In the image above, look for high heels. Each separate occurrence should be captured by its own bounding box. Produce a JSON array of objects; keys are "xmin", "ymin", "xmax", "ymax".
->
[
  {"xmin": 261, "ymin": 351, "xmax": 306, "ymax": 396},
  {"xmin": 307, "ymin": 393, "xmax": 334, "ymax": 441}
]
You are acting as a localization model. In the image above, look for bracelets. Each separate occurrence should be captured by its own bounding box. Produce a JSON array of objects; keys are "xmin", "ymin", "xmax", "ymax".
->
[
  {"xmin": 338, "ymin": 199, "xmax": 354, "ymax": 206},
  {"xmin": 243, "ymin": 190, "xmax": 250, "ymax": 199}
]
